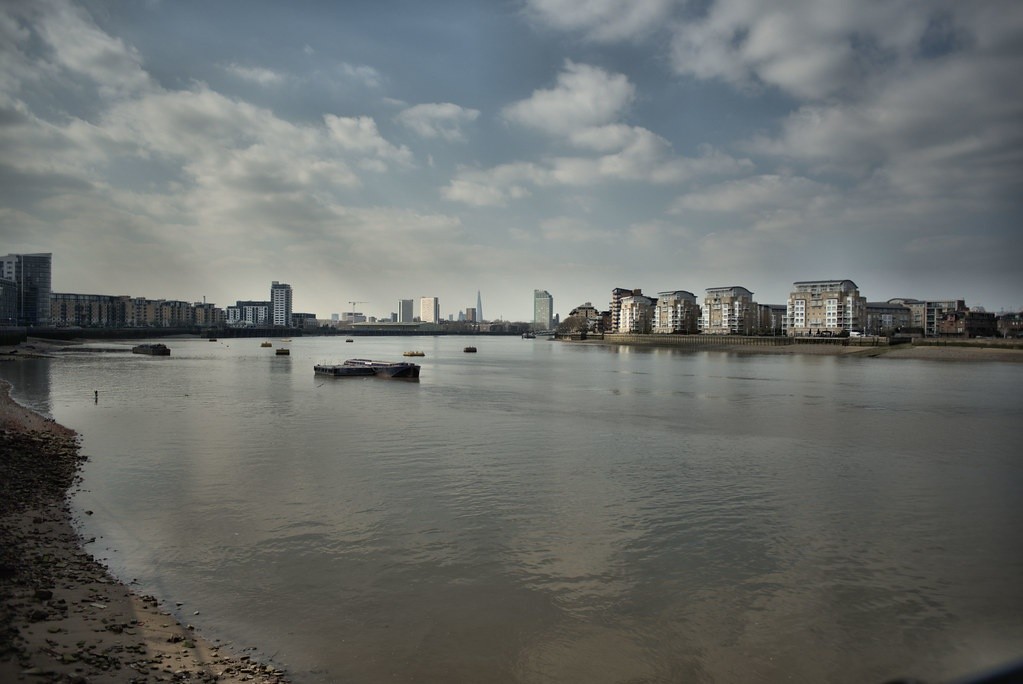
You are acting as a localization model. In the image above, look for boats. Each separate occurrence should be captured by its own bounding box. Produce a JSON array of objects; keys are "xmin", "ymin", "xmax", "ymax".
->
[
  {"xmin": 276, "ymin": 348, "xmax": 290, "ymax": 354},
  {"xmin": 522, "ymin": 331, "xmax": 536, "ymax": 339},
  {"xmin": 403, "ymin": 350, "xmax": 426, "ymax": 357},
  {"xmin": 463, "ymin": 347, "xmax": 477, "ymax": 352},
  {"xmin": 132, "ymin": 343, "xmax": 171, "ymax": 355},
  {"xmin": 313, "ymin": 358, "xmax": 422, "ymax": 379},
  {"xmin": 261, "ymin": 341, "xmax": 273, "ymax": 347}
]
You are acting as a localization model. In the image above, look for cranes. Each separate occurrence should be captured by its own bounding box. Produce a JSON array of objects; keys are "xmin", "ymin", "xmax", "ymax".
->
[{"xmin": 348, "ymin": 300, "xmax": 368, "ymax": 313}]
